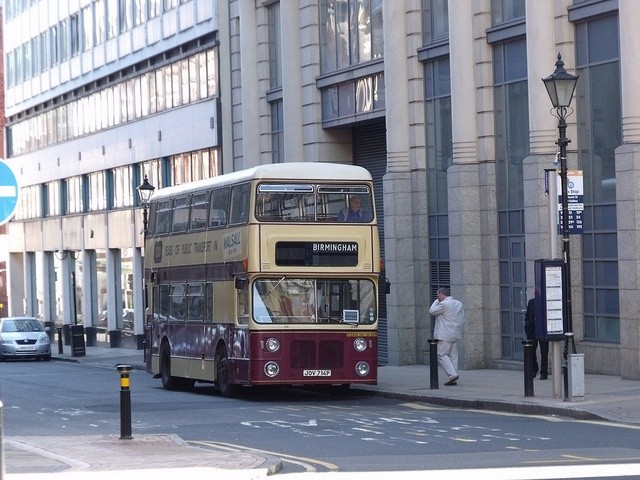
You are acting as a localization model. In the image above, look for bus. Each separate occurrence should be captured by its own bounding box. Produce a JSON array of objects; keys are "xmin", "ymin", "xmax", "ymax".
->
[{"xmin": 144, "ymin": 162, "xmax": 390, "ymax": 397}]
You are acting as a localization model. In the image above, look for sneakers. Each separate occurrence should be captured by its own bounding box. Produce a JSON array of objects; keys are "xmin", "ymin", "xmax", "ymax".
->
[
  {"xmin": 534, "ymin": 370, "xmax": 538, "ymax": 377},
  {"xmin": 444, "ymin": 375, "xmax": 458, "ymax": 385},
  {"xmin": 452, "ymin": 381, "xmax": 457, "ymax": 385},
  {"xmin": 540, "ymin": 374, "xmax": 547, "ymax": 379}
]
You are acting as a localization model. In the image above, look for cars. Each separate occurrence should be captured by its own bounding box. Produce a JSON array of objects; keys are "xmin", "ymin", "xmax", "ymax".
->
[{"xmin": 0, "ymin": 316, "xmax": 52, "ymax": 362}]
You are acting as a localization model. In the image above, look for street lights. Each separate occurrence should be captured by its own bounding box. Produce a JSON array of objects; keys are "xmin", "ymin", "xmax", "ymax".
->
[
  {"xmin": 542, "ymin": 52, "xmax": 580, "ymax": 402},
  {"xmin": 135, "ymin": 173, "xmax": 155, "ymax": 364}
]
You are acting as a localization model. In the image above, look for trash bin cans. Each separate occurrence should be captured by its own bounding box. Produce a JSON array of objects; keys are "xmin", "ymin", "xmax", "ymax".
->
[{"xmin": 69, "ymin": 325, "xmax": 85, "ymax": 356}]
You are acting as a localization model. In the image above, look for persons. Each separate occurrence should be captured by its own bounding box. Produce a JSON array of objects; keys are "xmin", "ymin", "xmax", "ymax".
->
[
  {"xmin": 525, "ymin": 298, "xmax": 549, "ymax": 381},
  {"xmin": 338, "ymin": 194, "xmax": 369, "ymax": 222},
  {"xmin": 429, "ymin": 287, "xmax": 464, "ymax": 386}
]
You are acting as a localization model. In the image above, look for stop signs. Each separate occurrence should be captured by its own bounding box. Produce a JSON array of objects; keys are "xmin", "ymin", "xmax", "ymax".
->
[{"xmin": 0, "ymin": 156, "xmax": 19, "ymax": 228}]
